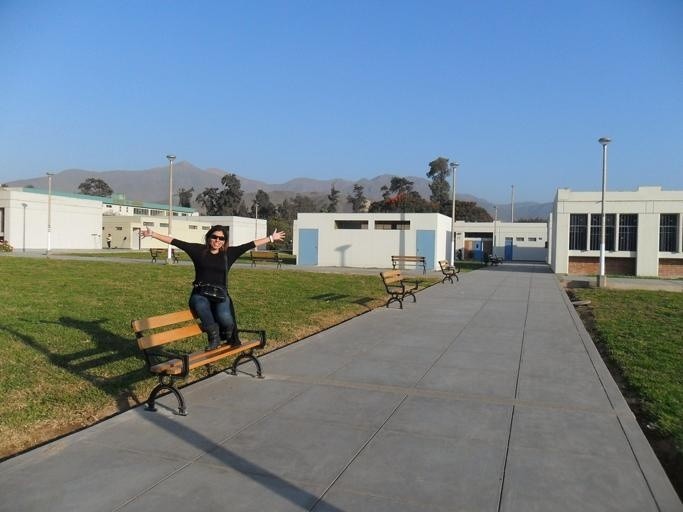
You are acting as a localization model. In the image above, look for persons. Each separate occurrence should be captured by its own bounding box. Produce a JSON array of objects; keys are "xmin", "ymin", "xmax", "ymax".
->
[
  {"xmin": 105, "ymin": 233, "xmax": 111, "ymax": 249},
  {"xmin": 136, "ymin": 224, "xmax": 285, "ymax": 352}
]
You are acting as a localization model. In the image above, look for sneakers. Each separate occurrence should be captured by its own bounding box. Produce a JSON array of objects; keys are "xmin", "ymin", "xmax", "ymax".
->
[{"xmin": 204, "ymin": 346, "xmax": 214, "ymax": 375}]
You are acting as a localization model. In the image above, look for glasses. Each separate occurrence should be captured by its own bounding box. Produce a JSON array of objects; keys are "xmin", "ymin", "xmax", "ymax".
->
[{"xmin": 208, "ymin": 234, "xmax": 225, "ymax": 241}]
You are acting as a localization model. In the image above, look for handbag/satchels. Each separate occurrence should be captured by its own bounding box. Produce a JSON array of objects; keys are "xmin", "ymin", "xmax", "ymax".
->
[{"xmin": 194, "ymin": 281, "xmax": 226, "ymax": 303}]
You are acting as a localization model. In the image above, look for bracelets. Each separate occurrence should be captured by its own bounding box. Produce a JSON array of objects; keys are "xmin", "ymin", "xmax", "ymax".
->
[{"xmin": 269, "ymin": 234, "xmax": 274, "ymax": 243}]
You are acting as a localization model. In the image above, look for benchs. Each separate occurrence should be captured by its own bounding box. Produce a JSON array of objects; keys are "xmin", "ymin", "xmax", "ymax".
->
[
  {"xmin": 150, "ymin": 248, "xmax": 180, "ymax": 263},
  {"xmin": 380, "ymin": 255, "xmax": 459, "ymax": 309},
  {"xmin": 130, "ymin": 309, "xmax": 266, "ymax": 415},
  {"xmin": 248, "ymin": 251, "xmax": 283, "ymax": 269}
]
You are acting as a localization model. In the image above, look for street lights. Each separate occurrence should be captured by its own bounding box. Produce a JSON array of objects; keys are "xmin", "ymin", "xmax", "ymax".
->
[
  {"xmin": 450, "ymin": 161, "xmax": 459, "ymax": 265},
  {"xmin": 47, "ymin": 171, "xmax": 54, "ymax": 256},
  {"xmin": 254, "ymin": 203, "xmax": 259, "ymax": 251},
  {"xmin": 598, "ymin": 137, "xmax": 611, "ymax": 276},
  {"xmin": 167, "ymin": 155, "xmax": 176, "ymax": 258},
  {"xmin": 493, "ymin": 185, "xmax": 515, "ymax": 223}
]
